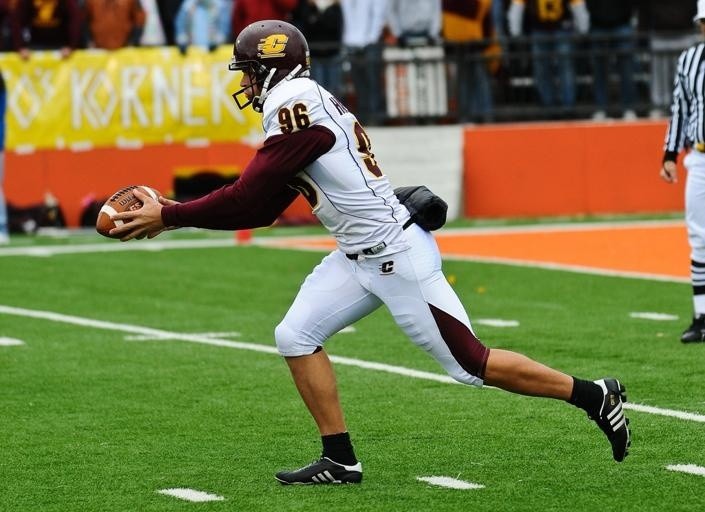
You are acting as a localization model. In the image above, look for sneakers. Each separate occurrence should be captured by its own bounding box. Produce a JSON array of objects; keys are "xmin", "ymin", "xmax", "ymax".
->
[
  {"xmin": 277, "ymin": 458, "xmax": 365, "ymax": 485},
  {"xmin": 681, "ymin": 315, "xmax": 704, "ymax": 342},
  {"xmin": 589, "ymin": 378, "xmax": 629, "ymax": 461}
]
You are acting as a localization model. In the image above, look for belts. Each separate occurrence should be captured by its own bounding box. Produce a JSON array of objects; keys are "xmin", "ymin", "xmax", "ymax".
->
[{"xmin": 346, "ymin": 241, "xmax": 389, "ymax": 260}]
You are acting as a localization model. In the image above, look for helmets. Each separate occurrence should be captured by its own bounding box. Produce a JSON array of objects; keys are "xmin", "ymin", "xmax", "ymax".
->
[{"xmin": 229, "ymin": 21, "xmax": 310, "ymax": 113}]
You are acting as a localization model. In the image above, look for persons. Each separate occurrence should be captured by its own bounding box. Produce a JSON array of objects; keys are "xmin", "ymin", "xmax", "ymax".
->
[
  {"xmin": 108, "ymin": 19, "xmax": 632, "ymax": 486},
  {"xmin": 657, "ymin": 0, "xmax": 705, "ymax": 344},
  {"xmin": 0, "ymin": 72, "xmax": 12, "ymax": 244},
  {"xmin": 0, "ymin": 0, "xmax": 698, "ymax": 123}
]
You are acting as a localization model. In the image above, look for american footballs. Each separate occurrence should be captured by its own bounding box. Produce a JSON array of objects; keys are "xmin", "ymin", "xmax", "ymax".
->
[{"xmin": 96, "ymin": 185, "xmax": 163, "ymax": 239}]
